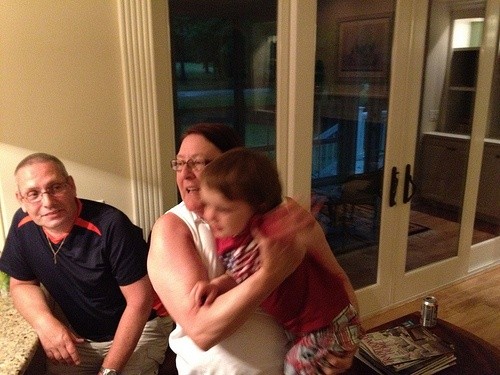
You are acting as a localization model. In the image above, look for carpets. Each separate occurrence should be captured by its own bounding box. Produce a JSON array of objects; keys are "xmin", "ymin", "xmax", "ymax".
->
[{"xmin": 325, "ymin": 194, "xmax": 432, "ymax": 259}]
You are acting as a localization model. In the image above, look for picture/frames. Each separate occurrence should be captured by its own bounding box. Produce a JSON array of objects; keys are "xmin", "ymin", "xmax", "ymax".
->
[{"xmin": 332, "ymin": 12, "xmax": 393, "ymax": 83}]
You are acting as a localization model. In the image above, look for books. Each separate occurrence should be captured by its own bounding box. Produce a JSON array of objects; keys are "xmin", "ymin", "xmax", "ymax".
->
[{"xmin": 357, "ymin": 319, "xmax": 458, "ymax": 375}]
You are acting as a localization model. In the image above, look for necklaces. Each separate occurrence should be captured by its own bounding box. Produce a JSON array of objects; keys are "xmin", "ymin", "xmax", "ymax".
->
[{"xmin": 42, "ymin": 208, "xmax": 75, "ymax": 265}]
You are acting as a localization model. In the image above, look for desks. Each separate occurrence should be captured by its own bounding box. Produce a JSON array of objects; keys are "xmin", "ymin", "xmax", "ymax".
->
[
  {"xmin": 347, "ymin": 308, "xmax": 500, "ymax": 375},
  {"xmin": 0, "ymin": 263, "xmax": 46, "ymax": 375}
]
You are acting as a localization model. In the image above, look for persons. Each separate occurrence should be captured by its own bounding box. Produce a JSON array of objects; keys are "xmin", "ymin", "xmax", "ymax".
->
[
  {"xmin": 0, "ymin": 153, "xmax": 174, "ymax": 375},
  {"xmin": 189, "ymin": 147, "xmax": 365, "ymax": 375},
  {"xmin": 147, "ymin": 122, "xmax": 363, "ymax": 375}
]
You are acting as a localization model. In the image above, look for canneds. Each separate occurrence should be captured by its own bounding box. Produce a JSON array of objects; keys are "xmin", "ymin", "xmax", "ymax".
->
[{"xmin": 420, "ymin": 295, "xmax": 438, "ymax": 327}]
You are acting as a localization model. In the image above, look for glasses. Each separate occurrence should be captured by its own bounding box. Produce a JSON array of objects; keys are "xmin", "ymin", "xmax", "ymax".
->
[
  {"xmin": 19, "ymin": 181, "xmax": 72, "ymax": 203},
  {"xmin": 169, "ymin": 158, "xmax": 214, "ymax": 171}
]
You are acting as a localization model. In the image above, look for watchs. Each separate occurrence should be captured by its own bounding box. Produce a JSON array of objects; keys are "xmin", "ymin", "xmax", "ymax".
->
[{"xmin": 100, "ymin": 366, "xmax": 117, "ymax": 375}]
